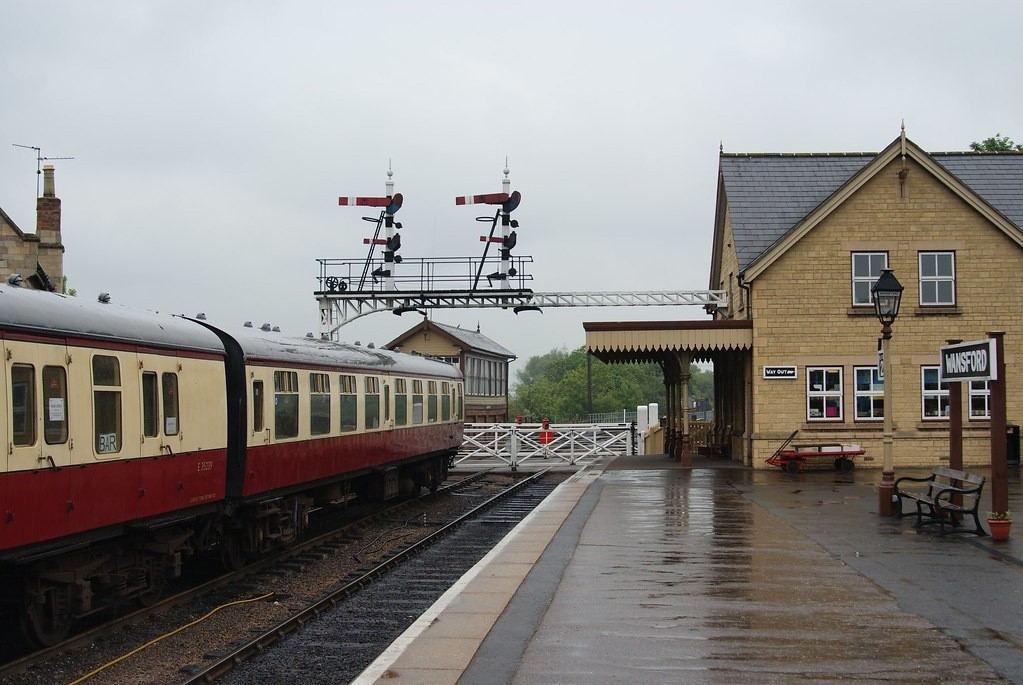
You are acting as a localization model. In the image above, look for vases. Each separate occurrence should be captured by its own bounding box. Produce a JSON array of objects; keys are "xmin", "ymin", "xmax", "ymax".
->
[{"xmin": 988, "ymin": 519, "xmax": 1012, "ymax": 540}]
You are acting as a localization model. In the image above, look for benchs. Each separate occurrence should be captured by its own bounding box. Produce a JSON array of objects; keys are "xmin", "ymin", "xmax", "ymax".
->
[{"xmin": 894, "ymin": 466, "xmax": 990, "ymax": 538}]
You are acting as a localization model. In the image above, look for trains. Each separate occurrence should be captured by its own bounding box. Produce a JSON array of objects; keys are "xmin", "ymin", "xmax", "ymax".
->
[{"xmin": 1, "ymin": 262, "xmax": 465, "ymax": 641}]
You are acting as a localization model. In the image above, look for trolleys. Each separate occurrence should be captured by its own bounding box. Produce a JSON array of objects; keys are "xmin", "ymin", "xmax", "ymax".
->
[{"xmin": 763, "ymin": 430, "xmax": 866, "ymax": 475}]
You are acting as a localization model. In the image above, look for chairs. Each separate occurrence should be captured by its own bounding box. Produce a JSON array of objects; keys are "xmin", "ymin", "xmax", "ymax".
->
[{"xmin": 706, "ymin": 425, "xmax": 732, "ymax": 460}]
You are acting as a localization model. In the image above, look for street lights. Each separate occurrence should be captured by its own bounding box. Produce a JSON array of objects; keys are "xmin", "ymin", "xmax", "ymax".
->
[{"xmin": 870, "ymin": 268, "xmax": 905, "ymax": 519}]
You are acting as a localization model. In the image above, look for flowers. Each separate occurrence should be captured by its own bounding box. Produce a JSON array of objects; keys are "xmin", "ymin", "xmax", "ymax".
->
[{"xmin": 986, "ymin": 508, "xmax": 1012, "ymax": 520}]
